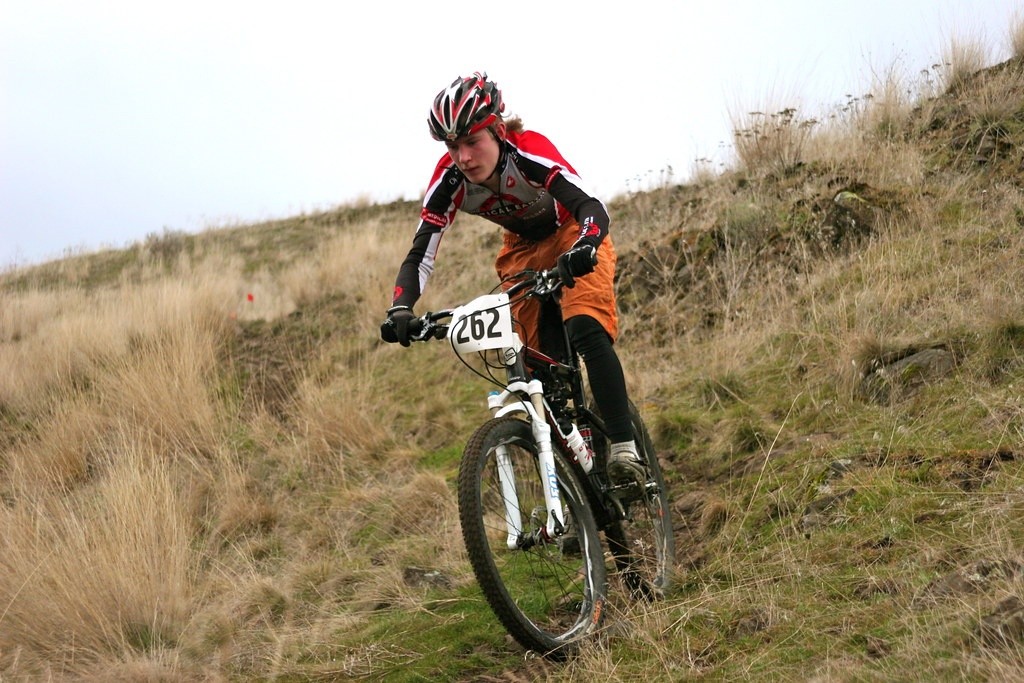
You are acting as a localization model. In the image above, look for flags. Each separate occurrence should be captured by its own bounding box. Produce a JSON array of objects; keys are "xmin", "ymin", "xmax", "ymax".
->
[{"xmin": 248, "ymin": 294, "xmax": 253, "ymax": 301}]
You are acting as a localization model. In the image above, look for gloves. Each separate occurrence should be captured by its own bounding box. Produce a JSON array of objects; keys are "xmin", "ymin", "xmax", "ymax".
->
[
  {"xmin": 380, "ymin": 309, "xmax": 418, "ymax": 348},
  {"xmin": 557, "ymin": 242, "xmax": 597, "ymax": 288}
]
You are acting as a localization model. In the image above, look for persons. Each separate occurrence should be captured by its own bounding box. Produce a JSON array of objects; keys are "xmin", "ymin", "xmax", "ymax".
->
[{"xmin": 380, "ymin": 72, "xmax": 650, "ymax": 554}]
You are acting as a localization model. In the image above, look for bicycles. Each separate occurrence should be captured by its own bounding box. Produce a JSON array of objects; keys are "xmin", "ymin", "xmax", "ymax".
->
[{"xmin": 406, "ymin": 255, "xmax": 678, "ymax": 663}]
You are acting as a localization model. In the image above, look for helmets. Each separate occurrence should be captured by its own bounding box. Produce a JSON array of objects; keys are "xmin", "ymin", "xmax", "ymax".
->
[{"xmin": 428, "ymin": 72, "xmax": 503, "ymax": 141}]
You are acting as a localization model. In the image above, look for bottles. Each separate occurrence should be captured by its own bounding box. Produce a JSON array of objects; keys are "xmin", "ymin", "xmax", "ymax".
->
[{"xmin": 557, "ymin": 418, "xmax": 592, "ymax": 473}]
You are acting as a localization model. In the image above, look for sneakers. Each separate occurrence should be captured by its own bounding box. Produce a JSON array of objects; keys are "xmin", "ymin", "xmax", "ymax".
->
[
  {"xmin": 606, "ymin": 451, "xmax": 645, "ymax": 500},
  {"xmin": 559, "ymin": 509, "xmax": 583, "ymax": 554}
]
